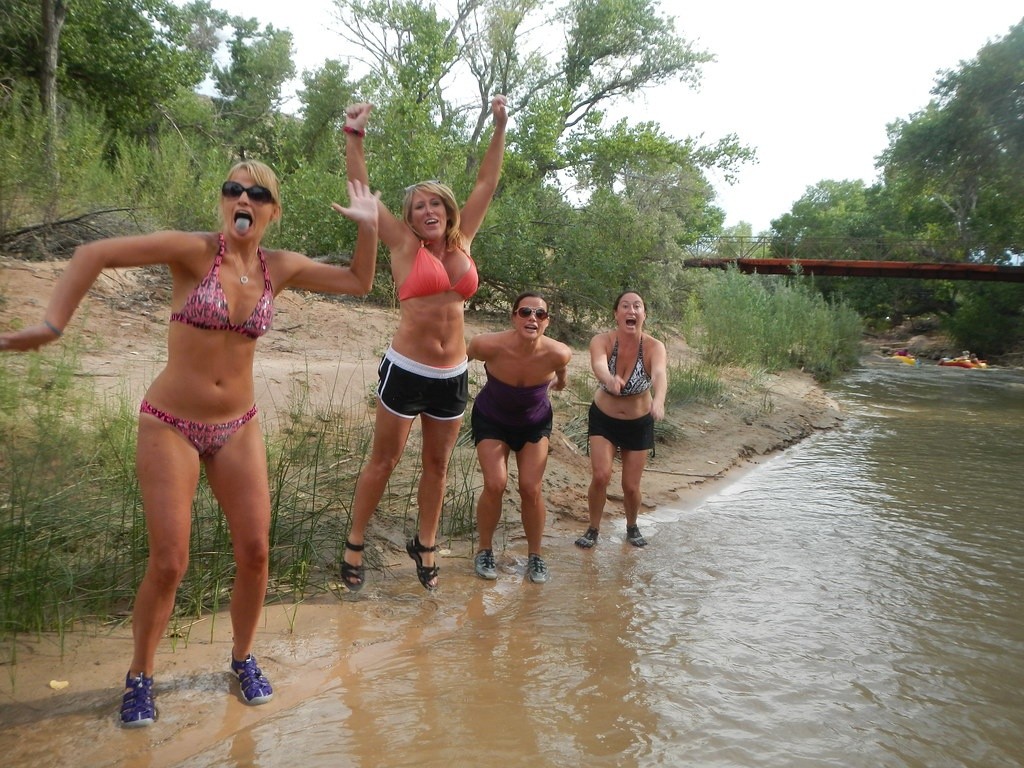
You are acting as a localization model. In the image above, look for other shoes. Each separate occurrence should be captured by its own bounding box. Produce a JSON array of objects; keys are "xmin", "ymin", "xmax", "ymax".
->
[
  {"xmin": 627, "ymin": 525, "xmax": 647, "ymax": 546},
  {"xmin": 575, "ymin": 526, "xmax": 599, "ymax": 549}
]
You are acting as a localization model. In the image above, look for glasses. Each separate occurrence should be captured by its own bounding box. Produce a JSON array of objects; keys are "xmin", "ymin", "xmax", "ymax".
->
[
  {"xmin": 514, "ymin": 306, "xmax": 549, "ymax": 321},
  {"xmin": 405, "ymin": 180, "xmax": 441, "ymax": 196},
  {"xmin": 222, "ymin": 181, "xmax": 276, "ymax": 204}
]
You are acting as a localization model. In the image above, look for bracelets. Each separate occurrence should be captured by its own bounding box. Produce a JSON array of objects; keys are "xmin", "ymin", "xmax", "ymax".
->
[
  {"xmin": 43, "ymin": 320, "xmax": 63, "ymax": 337},
  {"xmin": 343, "ymin": 126, "xmax": 367, "ymax": 138}
]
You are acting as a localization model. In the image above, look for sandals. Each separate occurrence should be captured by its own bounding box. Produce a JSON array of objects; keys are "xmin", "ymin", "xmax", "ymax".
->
[
  {"xmin": 340, "ymin": 539, "xmax": 364, "ymax": 590},
  {"xmin": 230, "ymin": 637, "xmax": 274, "ymax": 705},
  {"xmin": 120, "ymin": 670, "xmax": 156, "ymax": 727},
  {"xmin": 406, "ymin": 535, "xmax": 440, "ymax": 591}
]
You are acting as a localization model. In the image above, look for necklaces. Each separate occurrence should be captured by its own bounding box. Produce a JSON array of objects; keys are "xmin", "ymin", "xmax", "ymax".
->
[{"xmin": 223, "ymin": 240, "xmax": 259, "ymax": 285}]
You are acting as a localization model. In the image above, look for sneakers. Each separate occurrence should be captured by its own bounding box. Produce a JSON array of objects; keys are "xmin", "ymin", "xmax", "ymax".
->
[
  {"xmin": 528, "ymin": 553, "xmax": 549, "ymax": 584},
  {"xmin": 475, "ymin": 549, "xmax": 497, "ymax": 579}
]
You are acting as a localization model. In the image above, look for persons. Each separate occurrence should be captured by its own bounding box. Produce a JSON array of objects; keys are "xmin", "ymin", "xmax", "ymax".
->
[
  {"xmin": 340, "ymin": 93, "xmax": 510, "ymax": 593},
  {"xmin": 0, "ymin": 160, "xmax": 384, "ymax": 728},
  {"xmin": 467, "ymin": 290, "xmax": 573, "ymax": 586},
  {"xmin": 893, "ymin": 349, "xmax": 915, "ymax": 359},
  {"xmin": 575, "ymin": 290, "xmax": 668, "ymax": 548},
  {"xmin": 937, "ymin": 350, "xmax": 970, "ymax": 365},
  {"xmin": 970, "ymin": 353, "xmax": 987, "ymax": 364}
]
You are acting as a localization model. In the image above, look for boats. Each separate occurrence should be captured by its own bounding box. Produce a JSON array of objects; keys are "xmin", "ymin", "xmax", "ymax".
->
[
  {"xmin": 877, "ymin": 355, "xmax": 916, "ymax": 366},
  {"xmin": 938, "ymin": 358, "xmax": 987, "ymax": 370}
]
